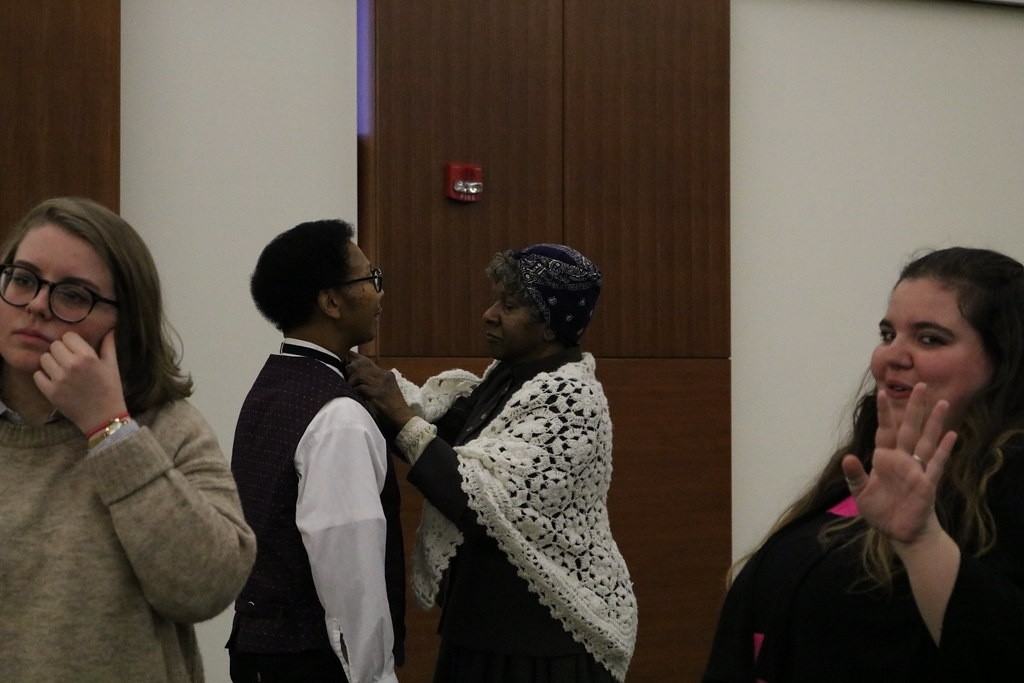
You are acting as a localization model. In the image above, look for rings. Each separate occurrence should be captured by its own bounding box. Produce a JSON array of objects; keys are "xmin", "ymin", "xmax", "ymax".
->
[{"xmin": 913, "ymin": 453, "xmax": 926, "ymax": 464}]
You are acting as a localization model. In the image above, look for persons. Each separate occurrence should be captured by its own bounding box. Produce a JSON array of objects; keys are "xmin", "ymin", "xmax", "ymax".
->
[
  {"xmin": 227, "ymin": 218, "xmax": 407, "ymax": 683},
  {"xmin": 0, "ymin": 195, "xmax": 257, "ymax": 683},
  {"xmin": 702, "ymin": 246, "xmax": 1024, "ymax": 683},
  {"xmin": 344, "ymin": 242, "xmax": 636, "ymax": 683}
]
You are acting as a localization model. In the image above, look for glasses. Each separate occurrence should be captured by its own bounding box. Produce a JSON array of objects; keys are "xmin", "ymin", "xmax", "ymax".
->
[
  {"xmin": 0, "ymin": 265, "xmax": 126, "ymax": 324},
  {"xmin": 340, "ymin": 267, "xmax": 384, "ymax": 293}
]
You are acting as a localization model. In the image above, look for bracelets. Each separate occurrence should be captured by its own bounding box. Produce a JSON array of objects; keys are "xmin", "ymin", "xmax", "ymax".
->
[{"xmin": 88, "ymin": 413, "xmax": 132, "ymax": 448}]
